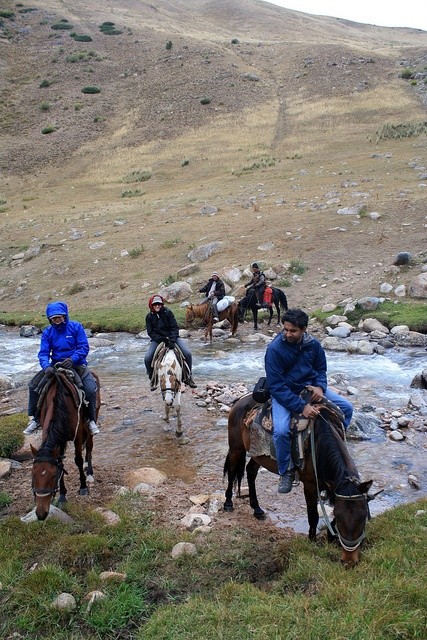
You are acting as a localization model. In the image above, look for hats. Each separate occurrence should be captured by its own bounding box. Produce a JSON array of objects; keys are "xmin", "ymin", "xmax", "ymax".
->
[
  {"xmin": 212, "ymin": 271, "xmax": 219, "ymax": 277},
  {"xmin": 49, "ymin": 315, "xmax": 63, "ymax": 319},
  {"xmin": 151, "ymin": 297, "xmax": 163, "ymax": 304},
  {"xmin": 252, "ymin": 263, "xmax": 259, "ymax": 268}
]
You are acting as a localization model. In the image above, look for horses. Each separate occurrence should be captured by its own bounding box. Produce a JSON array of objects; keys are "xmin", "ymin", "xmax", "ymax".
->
[
  {"xmin": 223, "ymin": 394, "xmax": 374, "ymax": 570},
  {"xmin": 237, "ymin": 287, "xmax": 288, "ymax": 330},
  {"xmin": 29, "ymin": 371, "xmax": 101, "ymax": 521},
  {"xmin": 158, "ymin": 350, "xmax": 186, "ymax": 438},
  {"xmin": 186, "ymin": 300, "xmax": 241, "ymax": 343}
]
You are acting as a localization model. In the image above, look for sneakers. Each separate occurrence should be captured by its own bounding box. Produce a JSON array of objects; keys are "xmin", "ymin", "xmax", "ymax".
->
[
  {"xmin": 256, "ymin": 303, "xmax": 261, "ymax": 306},
  {"xmin": 150, "ymin": 381, "xmax": 158, "ymax": 391},
  {"xmin": 22, "ymin": 420, "xmax": 38, "ymax": 435},
  {"xmin": 278, "ymin": 470, "xmax": 295, "ymax": 493},
  {"xmin": 184, "ymin": 379, "xmax": 197, "ymax": 388},
  {"xmin": 85, "ymin": 419, "xmax": 100, "ymax": 436},
  {"xmin": 213, "ymin": 317, "xmax": 220, "ymax": 321}
]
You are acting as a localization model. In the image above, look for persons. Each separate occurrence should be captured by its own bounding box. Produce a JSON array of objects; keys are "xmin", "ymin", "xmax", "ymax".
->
[
  {"xmin": 144, "ymin": 295, "xmax": 197, "ymax": 391},
  {"xmin": 23, "ymin": 302, "xmax": 100, "ymax": 436},
  {"xmin": 269, "ymin": 309, "xmax": 353, "ymax": 494},
  {"xmin": 197, "ymin": 272, "xmax": 224, "ymax": 321},
  {"xmin": 245, "ymin": 263, "xmax": 266, "ymax": 307}
]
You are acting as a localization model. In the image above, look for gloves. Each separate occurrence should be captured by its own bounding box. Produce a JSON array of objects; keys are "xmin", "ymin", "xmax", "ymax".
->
[
  {"xmin": 62, "ymin": 357, "xmax": 73, "ymax": 368},
  {"xmin": 162, "ymin": 337, "xmax": 168, "ymax": 343},
  {"xmin": 169, "ymin": 341, "xmax": 175, "ymax": 349},
  {"xmin": 245, "ymin": 284, "xmax": 248, "ymax": 288},
  {"xmin": 45, "ymin": 367, "xmax": 56, "ymax": 379}
]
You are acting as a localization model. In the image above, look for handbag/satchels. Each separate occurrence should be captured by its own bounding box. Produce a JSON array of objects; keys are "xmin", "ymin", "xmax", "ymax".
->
[{"xmin": 252, "ymin": 377, "xmax": 271, "ymax": 403}]
